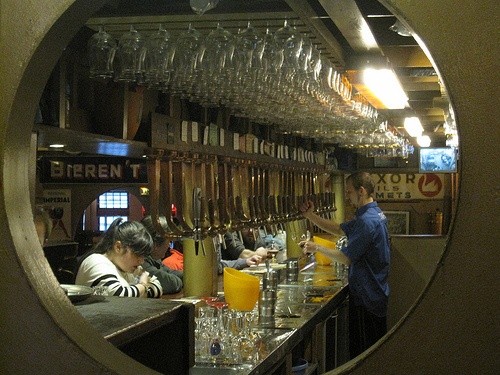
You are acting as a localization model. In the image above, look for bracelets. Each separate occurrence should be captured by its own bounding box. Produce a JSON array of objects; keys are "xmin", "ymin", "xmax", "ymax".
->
[{"xmin": 316, "ymin": 245, "xmax": 321, "ymax": 252}]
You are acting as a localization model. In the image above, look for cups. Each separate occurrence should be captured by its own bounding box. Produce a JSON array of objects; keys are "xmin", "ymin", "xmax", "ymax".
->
[
  {"xmin": 262, "ymin": 270, "xmax": 279, "ymax": 294},
  {"xmin": 285, "ymin": 260, "xmax": 298, "ymax": 283},
  {"xmin": 258, "ymin": 290, "xmax": 276, "ymax": 317},
  {"xmin": 296, "ymin": 234, "xmax": 315, "ymax": 257}
]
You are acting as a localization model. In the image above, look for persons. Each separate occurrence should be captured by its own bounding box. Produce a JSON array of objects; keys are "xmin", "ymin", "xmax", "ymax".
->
[
  {"xmin": 137, "ymin": 215, "xmax": 185, "ymax": 294},
  {"xmin": 33, "ymin": 206, "xmax": 52, "ymax": 246},
  {"xmin": 212, "ymin": 221, "xmax": 287, "ymax": 273},
  {"xmin": 73, "ymin": 216, "xmax": 161, "ymax": 299},
  {"xmin": 297, "ymin": 170, "xmax": 390, "ymax": 361}
]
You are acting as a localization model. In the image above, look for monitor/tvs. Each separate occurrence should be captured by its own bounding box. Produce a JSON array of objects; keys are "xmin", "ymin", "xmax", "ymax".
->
[{"xmin": 418, "ymin": 146, "xmax": 458, "ymax": 174}]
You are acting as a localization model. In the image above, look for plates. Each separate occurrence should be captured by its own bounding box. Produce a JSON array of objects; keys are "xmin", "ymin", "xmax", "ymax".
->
[{"xmin": 58, "ymin": 283, "xmax": 96, "ymax": 303}]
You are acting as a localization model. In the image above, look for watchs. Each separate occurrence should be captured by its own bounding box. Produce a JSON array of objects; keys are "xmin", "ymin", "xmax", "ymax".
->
[{"xmin": 138, "ymin": 281, "xmax": 149, "ymax": 293}]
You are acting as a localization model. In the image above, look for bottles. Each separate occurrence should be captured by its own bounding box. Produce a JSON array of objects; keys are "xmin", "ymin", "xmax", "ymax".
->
[{"xmin": 192, "ymin": 305, "xmax": 259, "ymax": 368}]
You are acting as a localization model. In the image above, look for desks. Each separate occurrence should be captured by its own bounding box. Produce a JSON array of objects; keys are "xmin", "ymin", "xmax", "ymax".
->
[{"xmin": 188, "ymin": 265, "xmax": 349, "ymax": 374}]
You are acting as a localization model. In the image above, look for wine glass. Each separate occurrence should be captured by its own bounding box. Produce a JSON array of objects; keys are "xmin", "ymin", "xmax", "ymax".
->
[{"xmin": 84, "ymin": 16, "xmax": 410, "ymax": 160}]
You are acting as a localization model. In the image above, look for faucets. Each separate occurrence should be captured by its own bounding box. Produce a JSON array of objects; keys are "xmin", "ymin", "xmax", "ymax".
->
[{"xmin": 195, "ymin": 191, "xmax": 338, "ymax": 256}]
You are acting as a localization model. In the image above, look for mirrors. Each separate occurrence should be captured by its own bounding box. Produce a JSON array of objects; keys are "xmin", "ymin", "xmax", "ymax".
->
[{"xmin": 29, "ymin": 0, "xmax": 462, "ymax": 375}]
